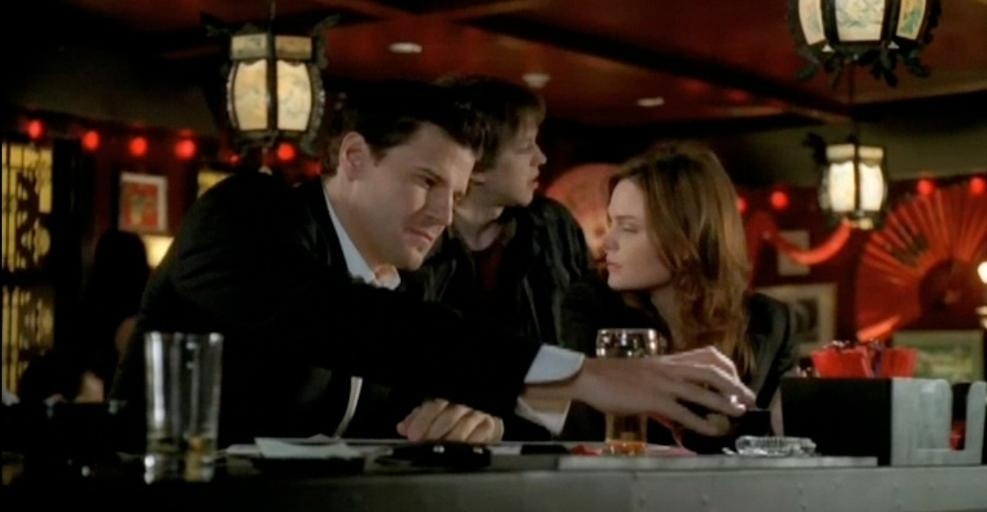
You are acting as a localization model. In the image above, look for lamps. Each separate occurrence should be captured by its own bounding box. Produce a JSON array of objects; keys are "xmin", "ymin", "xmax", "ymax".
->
[
  {"xmin": 794, "ymin": 0, "xmax": 931, "ymax": 57},
  {"xmin": 813, "ymin": 62, "xmax": 891, "ymax": 229},
  {"xmin": 198, "ymin": 2, "xmax": 336, "ymax": 153}
]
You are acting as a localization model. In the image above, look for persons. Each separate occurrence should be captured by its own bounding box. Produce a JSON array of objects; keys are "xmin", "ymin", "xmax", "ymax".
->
[
  {"xmin": 400, "ymin": 74, "xmax": 590, "ymax": 442},
  {"xmin": 95, "ymin": 71, "xmax": 756, "ymax": 464},
  {"xmin": 14, "ymin": 351, "xmax": 108, "ymax": 406},
  {"xmin": 50, "ymin": 201, "xmax": 151, "ymax": 347},
  {"xmin": 562, "ymin": 133, "xmax": 803, "ymax": 446}
]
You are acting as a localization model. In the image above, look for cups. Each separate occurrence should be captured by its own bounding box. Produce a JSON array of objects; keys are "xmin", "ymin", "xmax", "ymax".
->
[
  {"xmin": 594, "ymin": 330, "xmax": 657, "ymax": 452},
  {"xmin": 145, "ymin": 333, "xmax": 224, "ymax": 482}
]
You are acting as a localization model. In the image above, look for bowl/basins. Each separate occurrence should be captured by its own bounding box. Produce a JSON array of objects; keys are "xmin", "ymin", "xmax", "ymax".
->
[{"xmin": 2, "ymin": 396, "xmax": 106, "ymax": 477}]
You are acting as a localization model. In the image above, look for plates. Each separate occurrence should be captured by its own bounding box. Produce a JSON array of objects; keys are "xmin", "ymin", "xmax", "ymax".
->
[{"xmin": 226, "ymin": 440, "xmax": 393, "ymax": 474}]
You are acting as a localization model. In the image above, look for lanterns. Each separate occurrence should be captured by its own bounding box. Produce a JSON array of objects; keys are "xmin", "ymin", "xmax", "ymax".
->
[
  {"xmin": 192, "ymin": 11, "xmax": 345, "ymax": 161},
  {"xmin": 783, "ymin": 0, "xmax": 943, "ymax": 95},
  {"xmin": 801, "ymin": 129, "xmax": 894, "ymax": 230}
]
